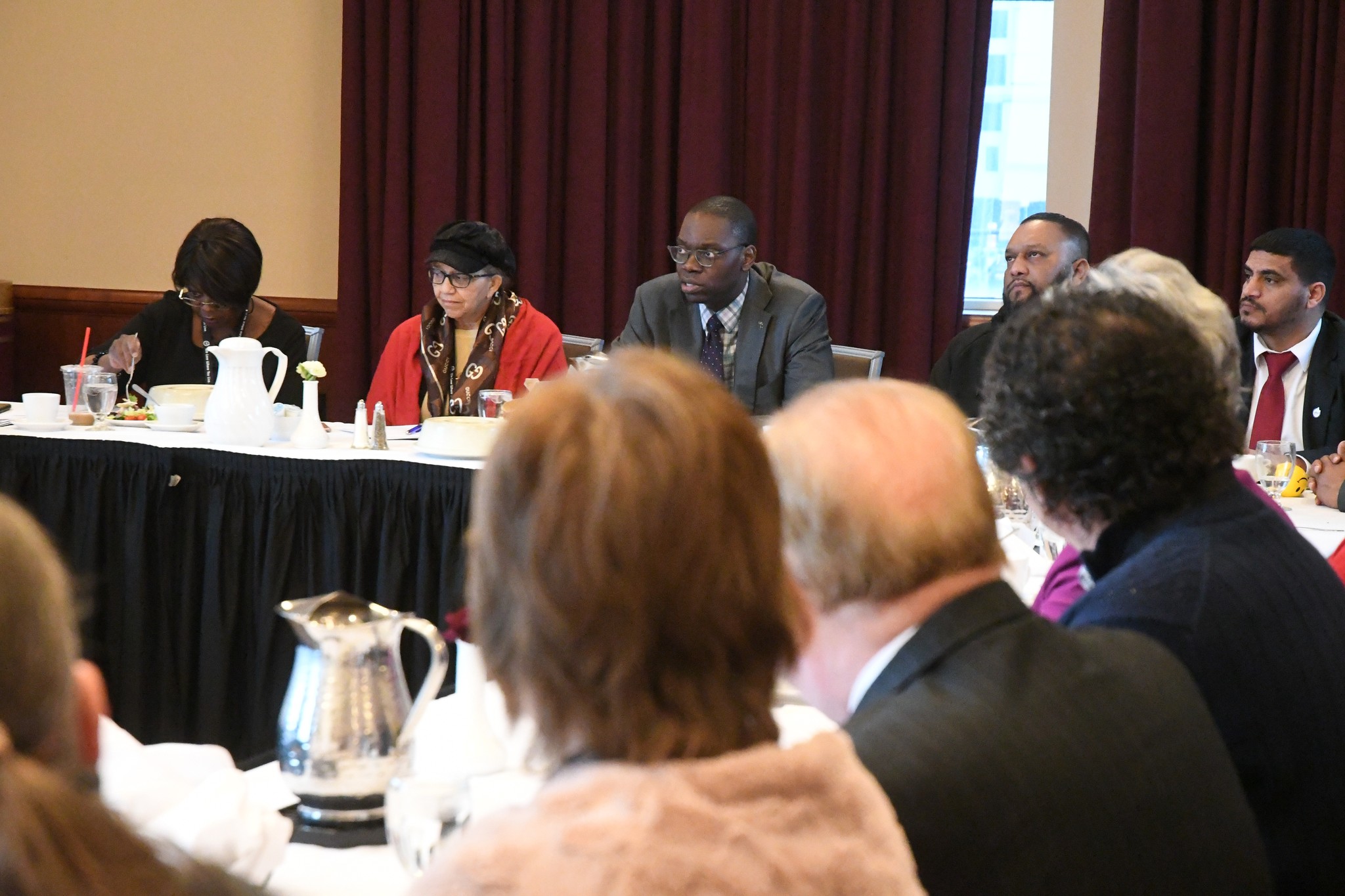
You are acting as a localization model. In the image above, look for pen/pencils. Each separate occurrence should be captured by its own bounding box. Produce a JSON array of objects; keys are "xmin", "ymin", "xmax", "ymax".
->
[{"xmin": 406, "ymin": 422, "xmax": 422, "ymax": 436}]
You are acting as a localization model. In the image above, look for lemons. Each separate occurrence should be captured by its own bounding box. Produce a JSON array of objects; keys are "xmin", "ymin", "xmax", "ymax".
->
[{"xmin": 1275, "ymin": 462, "xmax": 1309, "ymax": 497}]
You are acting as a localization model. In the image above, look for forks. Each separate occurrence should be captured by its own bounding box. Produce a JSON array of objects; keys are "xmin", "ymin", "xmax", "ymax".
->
[{"xmin": 126, "ymin": 332, "xmax": 138, "ymax": 400}]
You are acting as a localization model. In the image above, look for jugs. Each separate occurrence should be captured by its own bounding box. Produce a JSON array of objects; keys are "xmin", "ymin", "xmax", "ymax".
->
[
  {"xmin": 275, "ymin": 590, "xmax": 450, "ymax": 828},
  {"xmin": 204, "ymin": 337, "xmax": 288, "ymax": 446}
]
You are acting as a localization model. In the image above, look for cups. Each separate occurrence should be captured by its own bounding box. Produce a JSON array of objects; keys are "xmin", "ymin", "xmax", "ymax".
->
[
  {"xmin": 968, "ymin": 417, "xmax": 1032, "ymax": 523},
  {"xmin": 154, "ymin": 404, "xmax": 195, "ymax": 425},
  {"xmin": 22, "ymin": 393, "xmax": 60, "ymax": 423},
  {"xmin": 478, "ymin": 389, "xmax": 512, "ymax": 419},
  {"xmin": 60, "ymin": 365, "xmax": 104, "ymax": 426},
  {"xmin": 384, "ymin": 777, "xmax": 475, "ymax": 880}
]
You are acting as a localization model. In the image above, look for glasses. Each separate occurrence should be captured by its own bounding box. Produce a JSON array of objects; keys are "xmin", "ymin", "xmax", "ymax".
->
[
  {"xmin": 667, "ymin": 245, "xmax": 744, "ymax": 266},
  {"xmin": 179, "ymin": 286, "xmax": 230, "ymax": 312},
  {"xmin": 428, "ymin": 266, "xmax": 494, "ymax": 288}
]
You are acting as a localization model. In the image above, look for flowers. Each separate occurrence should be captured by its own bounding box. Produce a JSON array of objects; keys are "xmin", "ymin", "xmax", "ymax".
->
[{"xmin": 296, "ymin": 354, "xmax": 328, "ymax": 381}]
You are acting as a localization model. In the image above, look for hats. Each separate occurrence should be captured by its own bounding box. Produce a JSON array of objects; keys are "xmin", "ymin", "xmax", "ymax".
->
[{"xmin": 424, "ymin": 220, "xmax": 515, "ymax": 275}]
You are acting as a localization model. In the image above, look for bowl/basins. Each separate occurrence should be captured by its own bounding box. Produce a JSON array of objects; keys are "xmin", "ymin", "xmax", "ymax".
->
[
  {"xmin": 272, "ymin": 416, "xmax": 302, "ymax": 442},
  {"xmin": 1232, "ymin": 454, "xmax": 1269, "ymax": 482}
]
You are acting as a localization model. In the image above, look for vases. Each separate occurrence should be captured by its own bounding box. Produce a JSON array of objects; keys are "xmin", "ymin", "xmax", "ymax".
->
[{"xmin": 288, "ymin": 379, "xmax": 330, "ymax": 450}]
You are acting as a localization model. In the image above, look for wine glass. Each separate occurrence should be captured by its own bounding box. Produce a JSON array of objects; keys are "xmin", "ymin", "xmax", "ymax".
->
[
  {"xmin": 1255, "ymin": 440, "xmax": 1296, "ymax": 511},
  {"xmin": 82, "ymin": 372, "xmax": 118, "ymax": 431}
]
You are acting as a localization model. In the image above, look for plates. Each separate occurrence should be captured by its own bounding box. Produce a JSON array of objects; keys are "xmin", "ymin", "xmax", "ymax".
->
[
  {"xmin": 111, "ymin": 419, "xmax": 152, "ymax": 429},
  {"xmin": 145, "ymin": 421, "xmax": 200, "ymax": 432},
  {"xmin": 12, "ymin": 420, "xmax": 73, "ymax": 431}
]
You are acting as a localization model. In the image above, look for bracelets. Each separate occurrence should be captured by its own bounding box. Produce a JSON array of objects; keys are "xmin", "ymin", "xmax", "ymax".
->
[{"xmin": 91, "ymin": 351, "xmax": 122, "ymax": 379}]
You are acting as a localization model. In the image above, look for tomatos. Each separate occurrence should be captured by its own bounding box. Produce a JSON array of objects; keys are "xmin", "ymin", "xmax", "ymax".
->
[
  {"xmin": 125, "ymin": 416, "xmax": 138, "ymax": 420},
  {"xmin": 138, "ymin": 414, "xmax": 147, "ymax": 420}
]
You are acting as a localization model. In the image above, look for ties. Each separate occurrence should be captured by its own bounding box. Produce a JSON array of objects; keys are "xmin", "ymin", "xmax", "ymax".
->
[
  {"xmin": 701, "ymin": 313, "xmax": 726, "ymax": 383},
  {"xmin": 1248, "ymin": 350, "xmax": 1298, "ymax": 450}
]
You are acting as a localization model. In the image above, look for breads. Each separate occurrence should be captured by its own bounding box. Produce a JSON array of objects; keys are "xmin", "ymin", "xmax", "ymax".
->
[{"xmin": 69, "ymin": 411, "xmax": 94, "ymax": 425}]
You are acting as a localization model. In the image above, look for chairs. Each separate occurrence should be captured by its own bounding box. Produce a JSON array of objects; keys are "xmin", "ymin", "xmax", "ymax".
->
[
  {"xmin": 557, "ymin": 334, "xmax": 606, "ymax": 362},
  {"xmin": 828, "ymin": 342, "xmax": 886, "ymax": 382}
]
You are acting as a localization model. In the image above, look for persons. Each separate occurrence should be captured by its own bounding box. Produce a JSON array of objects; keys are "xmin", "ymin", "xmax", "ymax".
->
[
  {"xmin": 366, "ymin": 222, "xmax": 568, "ymax": 427},
  {"xmin": 1308, "ymin": 441, "xmax": 1345, "ymax": 512},
  {"xmin": 930, "ymin": 212, "xmax": 1092, "ymax": 418},
  {"xmin": 407, "ymin": 345, "xmax": 926, "ymax": 896},
  {"xmin": 1233, "ymin": 229, "xmax": 1345, "ymax": 475},
  {"xmin": 0, "ymin": 499, "xmax": 274, "ymax": 895},
  {"xmin": 81, "ymin": 218, "xmax": 325, "ymax": 421},
  {"xmin": 1029, "ymin": 246, "xmax": 1296, "ymax": 624},
  {"xmin": 763, "ymin": 379, "xmax": 1276, "ymax": 895},
  {"xmin": 982, "ymin": 286, "xmax": 1345, "ymax": 896},
  {"xmin": 609, "ymin": 195, "xmax": 835, "ymax": 429}
]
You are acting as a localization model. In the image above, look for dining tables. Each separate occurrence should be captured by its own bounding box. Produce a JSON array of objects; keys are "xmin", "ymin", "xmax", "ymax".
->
[{"xmin": 0, "ymin": 401, "xmax": 478, "ymax": 757}]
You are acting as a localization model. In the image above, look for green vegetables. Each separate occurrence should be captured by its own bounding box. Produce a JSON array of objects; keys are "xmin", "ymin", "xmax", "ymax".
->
[{"xmin": 108, "ymin": 393, "xmax": 158, "ymax": 421}]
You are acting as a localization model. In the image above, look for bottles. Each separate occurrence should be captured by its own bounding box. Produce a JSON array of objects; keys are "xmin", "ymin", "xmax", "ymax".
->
[
  {"xmin": 352, "ymin": 399, "xmax": 370, "ymax": 449},
  {"xmin": 370, "ymin": 401, "xmax": 389, "ymax": 451}
]
